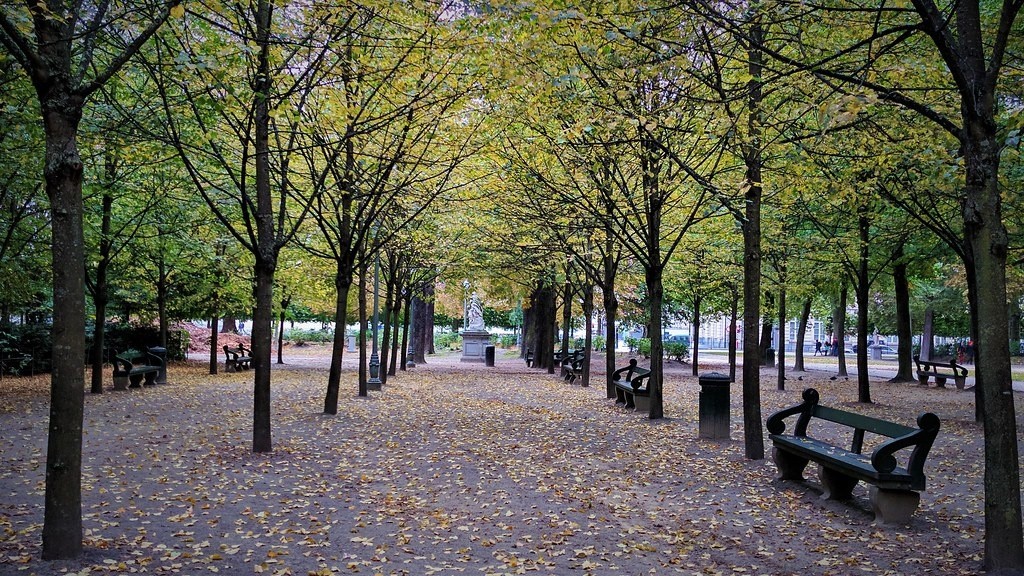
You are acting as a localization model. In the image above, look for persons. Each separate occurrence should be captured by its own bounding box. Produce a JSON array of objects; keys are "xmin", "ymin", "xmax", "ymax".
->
[
  {"xmin": 814, "ymin": 340, "xmax": 822, "ymax": 356},
  {"xmin": 468, "ymin": 292, "xmax": 483, "ymax": 324},
  {"xmin": 966, "ymin": 339, "xmax": 974, "ymax": 365},
  {"xmin": 832, "ymin": 337, "xmax": 838, "ymax": 356},
  {"xmin": 824, "ymin": 342, "xmax": 832, "ymax": 356},
  {"xmin": 239, "ymin": 321, "xmax": 244, "ymax": 331},
  {"xmin": 957, "ymin": 340, "xmax": 966, "ymax": 364}
]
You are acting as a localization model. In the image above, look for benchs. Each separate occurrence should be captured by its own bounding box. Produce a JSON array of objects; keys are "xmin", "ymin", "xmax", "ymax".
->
[
  {"xmin": 766, "ymin": 387, "xmax": 942, "ymax": 526},
  {"xmin": 913, "ymin": 353, "xmax": 968, "ymax": 390},
  {"xmin": 525, "ymin": 349, "xmax": 563, "ymax": 367},
  {"xmin": 612, "ymin": 359, "xmax": 651, "ymax": 412},
  {"xmin": 111, "ymin": 345, "xmax": 164, "ymax": 390},
  {"xmin": 556, "ymin": 347, "xmax": 585, "ymax": 366},
  {"xmin": 223, "ymin": 343, "xmax": 253, "ymax": 373},
  {"xmin": 562, "ymin": 350, "xmax": 585, "ymax": 384}
]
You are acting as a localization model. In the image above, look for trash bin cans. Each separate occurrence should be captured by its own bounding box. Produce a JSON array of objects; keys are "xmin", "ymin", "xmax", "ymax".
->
[
  {"xmin": 698, "ymin": 372, "xmax": 731, "ymax": 440},
  {"xmin": 766, "ymin": 348, "xmax": 775, "ymax": 367},
  {"xmin": 149, "ymin": 345, "xmax": 167, "ymax": 383}
]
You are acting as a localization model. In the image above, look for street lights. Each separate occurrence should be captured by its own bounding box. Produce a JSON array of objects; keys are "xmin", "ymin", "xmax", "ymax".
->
[
  {"xmin": 367, "ymin": 225, "xmax": 386, "ymax": 392},
  {"xmin": 463, "ymin": 279, "xmax": 469, "ymax": 331},
  {"xmin": 517, "ymin": 292, "xmax": 521, "ymax": 350}
]
